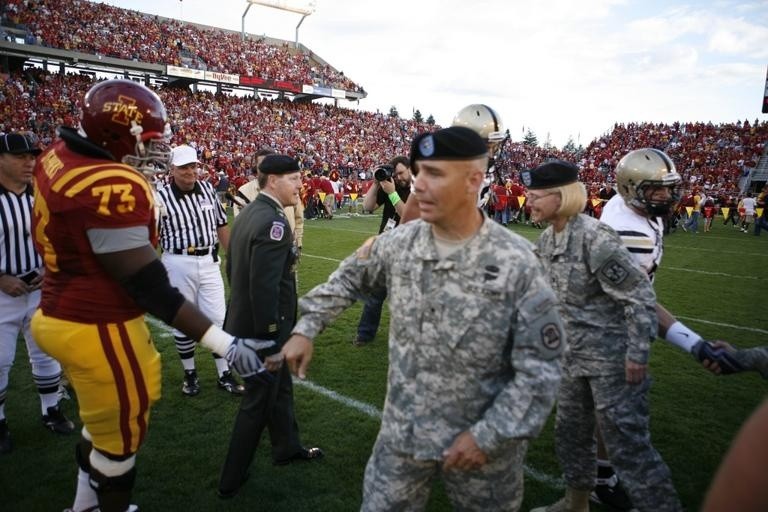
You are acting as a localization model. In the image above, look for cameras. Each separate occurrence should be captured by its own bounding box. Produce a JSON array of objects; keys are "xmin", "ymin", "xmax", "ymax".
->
[{"xmin": 375, "ymin": 163, "xmax": 395, "ymax": 182}]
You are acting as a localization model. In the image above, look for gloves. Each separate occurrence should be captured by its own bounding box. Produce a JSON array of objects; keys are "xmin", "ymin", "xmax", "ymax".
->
[
  {"xmin": 226, "ymin": 337, "xmax": 277, "ymax": 391},
  {"xmin": 691, "ymin": 339, "xmax": 747, "ymax": 374}
]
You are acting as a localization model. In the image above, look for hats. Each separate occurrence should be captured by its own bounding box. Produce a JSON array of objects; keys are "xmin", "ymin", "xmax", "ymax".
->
[
  {"xmin": 171, "ymin": 145, "xmax": 201, "ymax": 168},
  {"xmin": 0, "ymin": 133, "xmax": 43, "ymax": 153},
  {"xmin": 258, "ymin": 155, "xmax": 301, "ymax": 174},
  {"xmin": 520, "ymin": 159, "xmax": 577, "ymax": 189},
  {"xmin": 408, "ymin": 127, "xmax": 487, "ymax": 161}
]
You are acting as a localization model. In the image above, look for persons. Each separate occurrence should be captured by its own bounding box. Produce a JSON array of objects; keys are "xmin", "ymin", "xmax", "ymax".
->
[
  {"xmin": 352, "ymin": 155, "xmax": 412, "ymax": 348},
  {"xmin": 155, "ymin": 144, "xmax": 304, "ymax": 395},
  {"xmin": 282, "ymin": 125, "xmax": 567, "ymax": 511},
  {"xmin": 32, "ymin": 81, "xmax": 277, "ymax": 511},
  {"xmin": 519, "ymin": 149, "xmax": 767, "ymax": 511},
  {"xmin": 1, "ymin": 1, "xmax": 767, "ymax": 236},
  {"xmin": 0, "ymin": 132, "xmax": 75, "ymax": 445}
]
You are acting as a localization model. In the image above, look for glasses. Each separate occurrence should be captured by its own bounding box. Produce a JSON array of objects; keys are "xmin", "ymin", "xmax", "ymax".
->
[{"xmin": 525, "ymin": 193, "xmax": 554, "ymax": 199}]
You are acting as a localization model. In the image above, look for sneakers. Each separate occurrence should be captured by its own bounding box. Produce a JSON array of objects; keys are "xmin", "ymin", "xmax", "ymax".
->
[
  {"xmin": 42, "ymin": 403, "xmax": 74, "ymax": 433},
  {"xmin": 0, "ymin": 419, "xmax": 11, "ymax": 453},
  {"xmin": 182, "ymin": 369, "xmax": 201, "ymax": 397},
  {"xmin": 62, "ymin": 503, "xmax": 137, "ymax": 512},
  {"xmin": 218, "ymin": 369, "xmax": 245, "ymax": 394},
  {"xmin": 590, "ymin": 465, "xmax": 633, "ymax": 511}
]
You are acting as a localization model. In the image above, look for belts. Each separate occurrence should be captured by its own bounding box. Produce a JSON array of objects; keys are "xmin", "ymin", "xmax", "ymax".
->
[{"xmin": 174, "ymin": 249, "xmax": 209, "ymax": 256}]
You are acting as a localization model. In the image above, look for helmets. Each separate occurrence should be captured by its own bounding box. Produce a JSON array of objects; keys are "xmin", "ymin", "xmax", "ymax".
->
[
  {"xmin": 455, "ymin": 105, "xmax": 508, "ymax": 160},
  {"xmin": 73, "ymin": 79, "xmax": 175, "ymax": 185},
  {"xmin": 615, "ymin": 147, "xmax": 683, "ymax": 216}
]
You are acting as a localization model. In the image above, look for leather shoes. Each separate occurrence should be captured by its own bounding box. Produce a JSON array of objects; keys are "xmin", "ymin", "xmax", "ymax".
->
[{"xmin": 272, "ymin": 447, "xmax": 326, "ymax": 467}]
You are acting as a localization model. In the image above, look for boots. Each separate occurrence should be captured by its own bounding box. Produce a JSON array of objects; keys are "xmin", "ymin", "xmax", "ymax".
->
[{"xmin": 529, "ymin": 485, "xmax": 590, "ymax": 512}]
